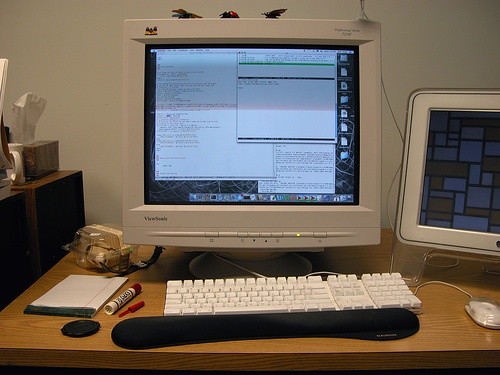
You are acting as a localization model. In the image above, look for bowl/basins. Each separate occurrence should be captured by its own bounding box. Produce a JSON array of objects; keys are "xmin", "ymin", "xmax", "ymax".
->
[{"xmin": 69, "ymin": 223, "xmax": 141, "ymax": 268}]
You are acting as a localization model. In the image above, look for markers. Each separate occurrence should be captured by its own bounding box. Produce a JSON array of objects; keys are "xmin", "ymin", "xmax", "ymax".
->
[{"xmin": 103, "ymin": 283, "xmax": 142, "ymax": 315}]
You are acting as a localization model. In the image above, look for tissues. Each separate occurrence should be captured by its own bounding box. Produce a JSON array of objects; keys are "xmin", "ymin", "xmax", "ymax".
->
[{"xmin": 9, "ymin": 92, "xmax": 59, "ymax": 181}]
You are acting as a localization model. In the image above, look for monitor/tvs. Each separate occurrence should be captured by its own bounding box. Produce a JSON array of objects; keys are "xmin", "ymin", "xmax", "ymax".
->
[
  {"xmin": 395, "ymin": 89, "xmax": 499, "ymax": 254},
  {"xmin": 121, "ymin": 20, "xmax": 381, "ymax": 278}
]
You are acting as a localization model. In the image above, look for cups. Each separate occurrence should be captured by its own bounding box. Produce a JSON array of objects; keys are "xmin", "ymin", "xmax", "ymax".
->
[{"xmin": 6, "ymin": 143, "xmax": 26, "ymax": 185}]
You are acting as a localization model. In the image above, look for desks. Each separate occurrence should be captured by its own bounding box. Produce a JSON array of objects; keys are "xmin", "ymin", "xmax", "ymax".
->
[
  {"xmin": 0, "ymin": 168, "xmax": 86, "ymax": 293},
  {"xmin": 0, "ymin": 227, "xmax": 499, "ymax": 375}
]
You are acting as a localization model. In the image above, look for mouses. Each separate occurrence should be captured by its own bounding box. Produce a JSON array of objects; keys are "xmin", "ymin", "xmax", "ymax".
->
[{"xmin": 465, "ymin": 297, "xmax": 500, "ymax": 329}]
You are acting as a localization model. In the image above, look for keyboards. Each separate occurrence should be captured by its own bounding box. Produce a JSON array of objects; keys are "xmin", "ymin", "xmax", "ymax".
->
[{"xmin": 165, "ymin": 272, "xmax": 422, "ymax": 315}]
